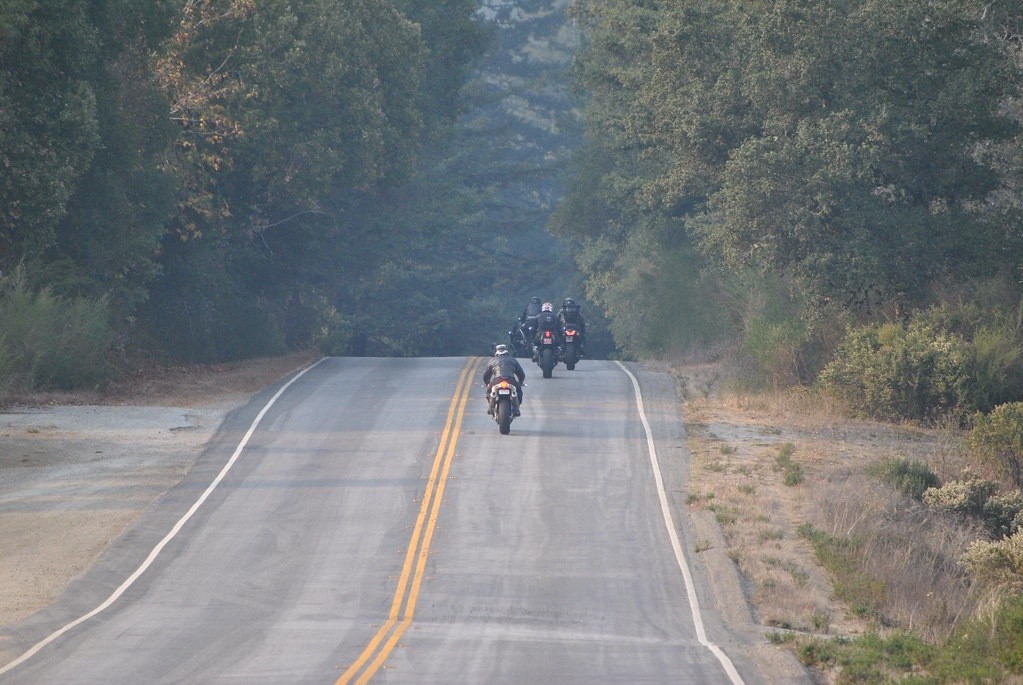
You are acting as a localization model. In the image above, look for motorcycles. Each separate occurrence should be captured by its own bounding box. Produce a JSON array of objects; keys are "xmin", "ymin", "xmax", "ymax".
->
[
  {"xmin": 487, "ymin": 381, "xmax": 527, "ymax": 434},
  {"xmin": 508, "ymin": 319, "xmax": 587, "ymax": 378}
]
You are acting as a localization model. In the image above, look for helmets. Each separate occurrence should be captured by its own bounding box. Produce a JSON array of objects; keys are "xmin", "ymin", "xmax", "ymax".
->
[
  {"xmin": 542, "ymin": 302, "xmax": 553, "ymax": 313},
  {"xmin": 496, "ymin": 344, "xmax": 508, "ymax": 355},
  {"xmin": 531, "ymin": 296, "xmax": 540, "ymax": 304},
  {"xmin": 563, "ymin": 298, "xmax": 575, "ymax": 307}
]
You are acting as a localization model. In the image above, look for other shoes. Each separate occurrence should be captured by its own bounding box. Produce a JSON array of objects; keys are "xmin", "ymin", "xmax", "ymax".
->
[
  {"xmin": 487, "ymin": 409, "xmax": 494, "ymax": 416},
  {"xmin": 532, "ymin": 351, "xmax": 542, "ymax": 361},
  {"xmin": 580, "ymin": 352, "xmax": 585, "ymax": 357},
  {"xmin": 513, "ymin": 410, "xmax": 521, "ymax": 417}
]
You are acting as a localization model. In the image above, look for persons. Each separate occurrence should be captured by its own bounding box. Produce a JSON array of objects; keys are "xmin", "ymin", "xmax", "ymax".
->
[
  {"xmin": 484, "ymin": 344, "xmax": 526, "ymax": 416},
  {"xmin": 491, "ymin": 296, "xmax": 585, "ymax": 362}
]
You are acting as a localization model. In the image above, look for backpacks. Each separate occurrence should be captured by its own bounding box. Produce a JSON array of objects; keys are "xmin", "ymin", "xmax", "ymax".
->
[
  {"xmin": 562, "ymin": 304, "xmax": 580, "ymax": 330},
  {"xmin": 540, "ymin": 312, "xmax": 553, "ymax": 330}
]
